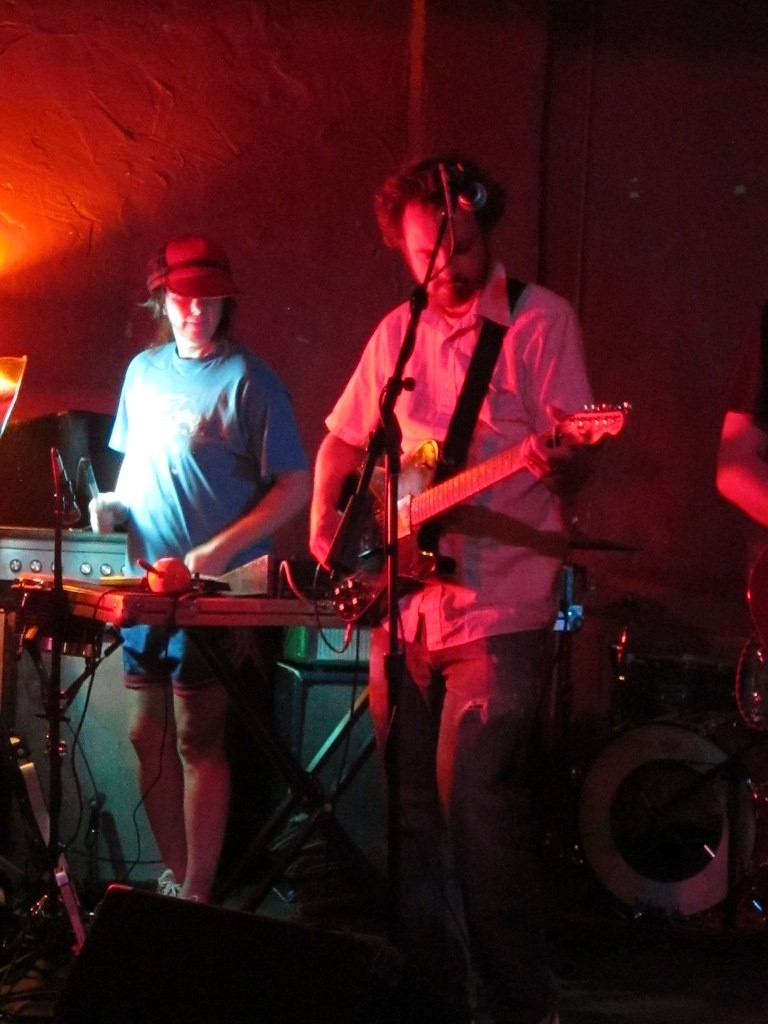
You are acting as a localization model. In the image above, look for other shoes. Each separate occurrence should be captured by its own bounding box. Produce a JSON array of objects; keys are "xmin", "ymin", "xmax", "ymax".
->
[{"xmin": 157, "ymin": 868, "xmax": 182, "ymax": 898}]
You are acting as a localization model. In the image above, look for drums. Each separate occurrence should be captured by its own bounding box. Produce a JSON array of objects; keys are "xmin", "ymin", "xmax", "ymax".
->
[
  {"xmin": 568, "ymin": 710, "xmax": 767, "ymax": 915},
  {"xmin": 734, "ymin": 637, "xmax": 768, "ymax": 731}
]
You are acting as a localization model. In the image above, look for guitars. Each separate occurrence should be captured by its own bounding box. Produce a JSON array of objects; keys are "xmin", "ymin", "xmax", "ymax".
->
[
  {"xmin": 170, "ymin": 563, "xmax": 467, "ymax": 993},
  {"xmin": 331, "ymin": 401, "xmax": 634, "ymax": 631}
]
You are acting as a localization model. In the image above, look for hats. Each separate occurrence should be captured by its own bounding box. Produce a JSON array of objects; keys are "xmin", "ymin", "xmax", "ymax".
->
[{"xmin": 147, "ymin": 237, "xmax": 236, "ymax": 300}]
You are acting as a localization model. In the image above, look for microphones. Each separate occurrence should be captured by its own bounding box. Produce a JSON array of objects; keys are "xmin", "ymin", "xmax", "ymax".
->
[
  {"xmin": 439, "ymin": 161, "xmax": 487, "ymax": 211},
  {"xmin": 51, "ymin": 448, "xmax": 81, "ymax": 526}
]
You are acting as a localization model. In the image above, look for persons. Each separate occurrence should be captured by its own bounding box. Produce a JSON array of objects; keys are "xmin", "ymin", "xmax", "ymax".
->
[
  {"xmin": 715, "ymin": 293, "xmax": 768, "ymax": 572},
  {"xmin": 88, "ymin": 236, "xmax": 312, "ymax": 904},
  {"xmin": 310, "ymin": 153, "xmax": 597, "ymax": 1024}
]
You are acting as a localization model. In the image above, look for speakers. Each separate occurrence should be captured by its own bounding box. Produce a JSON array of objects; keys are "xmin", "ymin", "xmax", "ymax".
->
[
  {"xmin": 55, "ymin": 887, "xmax": 476, "ymax": 1024},
  {"xmin": 274, "ymin": 663, "xmax": 391, "ymax": 885}
]
